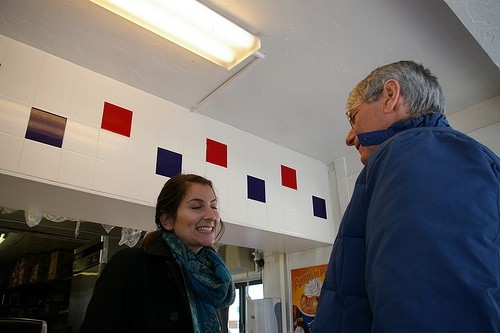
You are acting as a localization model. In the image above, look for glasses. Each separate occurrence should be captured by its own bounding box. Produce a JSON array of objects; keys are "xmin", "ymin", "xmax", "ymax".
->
[{"xmin": 345, "ymin": 89, "xmax": 384, "ymax": 130}]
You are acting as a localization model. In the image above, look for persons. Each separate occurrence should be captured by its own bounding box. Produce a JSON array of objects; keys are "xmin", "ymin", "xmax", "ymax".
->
[
  {"xmin": 307, "ymin": 60, "xmax": 500, "ymax": 333},
  {"xmin": 78, "ymin": 173, "xmax": 235, "ymax": 333}
]
